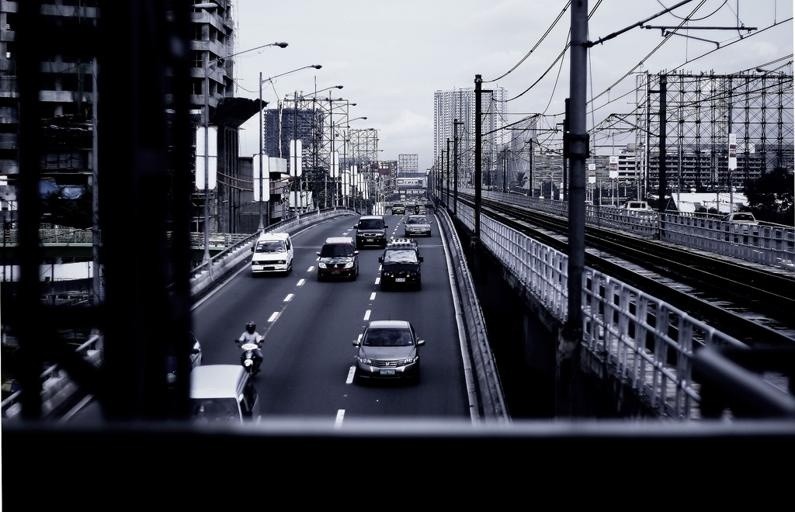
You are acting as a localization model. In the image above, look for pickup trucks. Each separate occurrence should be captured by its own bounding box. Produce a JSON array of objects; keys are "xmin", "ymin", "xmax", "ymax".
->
[{"xmin": 617, "ymin": 200, "xmax": 659, "ymax": 226}]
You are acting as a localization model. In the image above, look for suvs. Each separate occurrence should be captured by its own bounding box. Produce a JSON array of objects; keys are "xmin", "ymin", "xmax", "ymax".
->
[
  {"xmin": 250, "ymin": 232, "xmax": 294, "ymax": 277},
  {"xmin": 352, "ymin": 215, "xmax": 388, "ymax": 249},
  {"xmin": 314, "ymin": 237, "xmax": 360, "ymax": 282},
  {"xmin": 721, "ymin": 212, "xmax": 760, "ymax": 243}
]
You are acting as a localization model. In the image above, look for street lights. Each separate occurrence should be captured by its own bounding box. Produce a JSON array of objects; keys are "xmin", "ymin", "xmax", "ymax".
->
[
  {"xmin": 201, "ymin": 42, "xmax": 290, "ymax": 258},
  {"xmin": 315, "ymin": 103, "xmax": 386, "ymax": 216},
  {"xmin": 293, "ymin": 84, "xmax": 343, "ymax": 218},
  {"xmin": 257, "ymin": 64, "xmax": 323, "ymax": 231}
]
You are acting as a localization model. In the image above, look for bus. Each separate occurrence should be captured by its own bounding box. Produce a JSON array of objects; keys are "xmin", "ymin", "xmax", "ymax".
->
[{"xmin": 414, "ymin": 204, "xmax": 427, "ymax": 214}]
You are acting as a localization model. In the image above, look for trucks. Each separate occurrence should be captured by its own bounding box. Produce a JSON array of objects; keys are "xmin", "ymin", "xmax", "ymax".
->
[{"xmin": 377, "ymin": 238, "xmax": 424, "ymax": 291}]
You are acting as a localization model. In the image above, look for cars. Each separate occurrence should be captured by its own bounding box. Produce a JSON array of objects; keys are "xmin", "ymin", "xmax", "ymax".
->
[
  {"xmin": 391, "ymin": 203, "xmax": 406, "ymax": 215},
  {"xmin": 418, "ymin": 209, "xmax": 426, "ymax": 214},
  {"xmin": 350, "ymin": 319, "xmax": 425, "ymax": 386},
  {"xmin": 191, "ymin": 334, "xmax": 203, "ymax": 370},
  {"xmin": 403, "ymin": 214, "xmax": 433, "ymax": 237}
]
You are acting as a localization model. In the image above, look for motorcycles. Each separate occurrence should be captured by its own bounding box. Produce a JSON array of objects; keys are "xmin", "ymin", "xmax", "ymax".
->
[{"xmin": 234, "ymin": 336, "xmax": 265, "ymax": 375}]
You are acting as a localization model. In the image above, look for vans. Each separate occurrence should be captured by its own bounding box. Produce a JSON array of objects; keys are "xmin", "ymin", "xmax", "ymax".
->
[{"xmin": 189, "ymin": 364, "xmax": 262, "ymax": 426}]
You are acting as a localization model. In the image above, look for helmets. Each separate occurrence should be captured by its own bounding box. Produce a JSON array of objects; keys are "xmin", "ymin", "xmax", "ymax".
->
[{"xmin": 245, "ymin": 321, "xmax": 256, "ymax": 331}]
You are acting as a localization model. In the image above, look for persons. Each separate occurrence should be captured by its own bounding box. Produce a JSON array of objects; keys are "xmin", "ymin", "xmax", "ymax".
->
[{"xmin": 237, "ymin": 320, "xmax": 265, "ymax": 372}]
